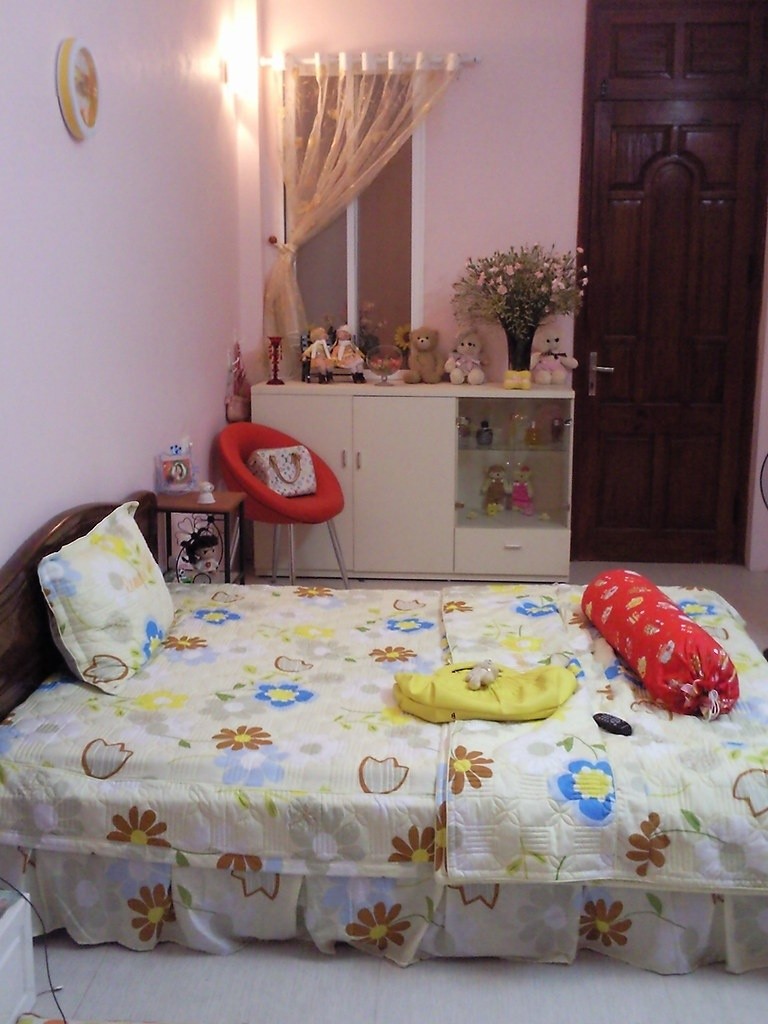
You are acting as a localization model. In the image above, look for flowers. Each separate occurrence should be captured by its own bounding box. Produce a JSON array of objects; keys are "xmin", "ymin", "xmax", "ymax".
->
[{"xmin": 449, "ymin": 239, "xmax": 586, "ymax": 341}]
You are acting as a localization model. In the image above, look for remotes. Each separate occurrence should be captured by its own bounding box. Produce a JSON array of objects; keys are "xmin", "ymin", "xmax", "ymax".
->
[{"xmin": 593, "ymin": 713, "xmax": 632, "ymax": 736}]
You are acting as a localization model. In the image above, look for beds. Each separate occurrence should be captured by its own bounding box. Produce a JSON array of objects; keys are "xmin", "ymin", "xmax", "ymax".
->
[{"xmin": 0, "ymin": 488, "xmax": 768, "ymax": 975}]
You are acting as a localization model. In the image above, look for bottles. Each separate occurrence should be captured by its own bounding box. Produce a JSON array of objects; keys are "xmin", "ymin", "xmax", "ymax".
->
[
  {"xmin": 457, "ymin": 416, "xmax": 471, "ymax": 447},
  {"xmin": 552, "ymin": 418, "xmax": 563, "ymax": 442},
  {"xmin": 527, "ymin": 421, "xmax": 539, "ymax": 445},
  {"xmin": 476, "ymin": 419, "xmax": 493, "ymax": 446}
]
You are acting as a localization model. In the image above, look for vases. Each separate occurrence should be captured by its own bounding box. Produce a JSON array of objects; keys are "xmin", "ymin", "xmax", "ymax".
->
[{"xmin": 497, "ymin": 308, "xmax": 540, "ymax": 371}]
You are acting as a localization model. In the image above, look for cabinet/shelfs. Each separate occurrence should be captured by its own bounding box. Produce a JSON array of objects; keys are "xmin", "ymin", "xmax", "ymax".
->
[{"xmin": 249, "ymin": 370, "xmax": 575, "ymax": 582}]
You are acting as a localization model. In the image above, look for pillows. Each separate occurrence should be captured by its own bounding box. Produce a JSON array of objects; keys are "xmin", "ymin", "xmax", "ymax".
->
[
  {"xmin": 37, "ymin": 501, "xmax": 175, "ymax": 694},
  {"xmin": 581, "ymin": 569, "xmax": 740, "ymax": 722}
]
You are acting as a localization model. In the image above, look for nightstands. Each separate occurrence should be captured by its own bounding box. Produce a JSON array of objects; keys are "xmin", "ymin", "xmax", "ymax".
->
[
  {"xmin": 0, "ymin": 891, "xmax": 39, "ymax": 1024},
  {"xmin": 155, "ymin": 492, "xmax": 247, "ymax": 586}
]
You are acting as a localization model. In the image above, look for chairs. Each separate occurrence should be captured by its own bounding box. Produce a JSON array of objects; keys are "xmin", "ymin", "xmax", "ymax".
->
[{"xmin": 217, "ymin": 422, "xmax": 351, "ymax": 590}]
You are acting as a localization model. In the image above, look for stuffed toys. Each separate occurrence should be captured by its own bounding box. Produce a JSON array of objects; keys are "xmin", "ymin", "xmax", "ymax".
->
[
  {"xmin": 403, "ymin": 326, "xmax": 446, "ymax": 384},
  {"xmin": 480, "ymin": 462, "xmax": 535, "ymax": 517},
  {"xmin": 300, "ymin": 326, "xmax": 332, "ymax": 385},
  {"xmin": 443, "ymin": 325, "xmax": 492, "ymax": 385},
  {"xmin": 529, "ymin": 321, "xmax": 579, "ymax": 386},
  {"xmin": 464, "ymin": 659, "xmax": 499, "ymax": 690},
  {"xmin": 330, "ymin": 324, "xmax": 368, "ymax": 384}
]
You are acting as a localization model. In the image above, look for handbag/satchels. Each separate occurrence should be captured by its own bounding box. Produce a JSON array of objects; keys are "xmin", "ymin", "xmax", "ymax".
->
[
  {"xmin": 247, "ymin": 445, "xmax": 317, "ymax": 496},
  {"xmin": 392, "ymin": 658, "xmax": 577, "ymax": 724}
]
